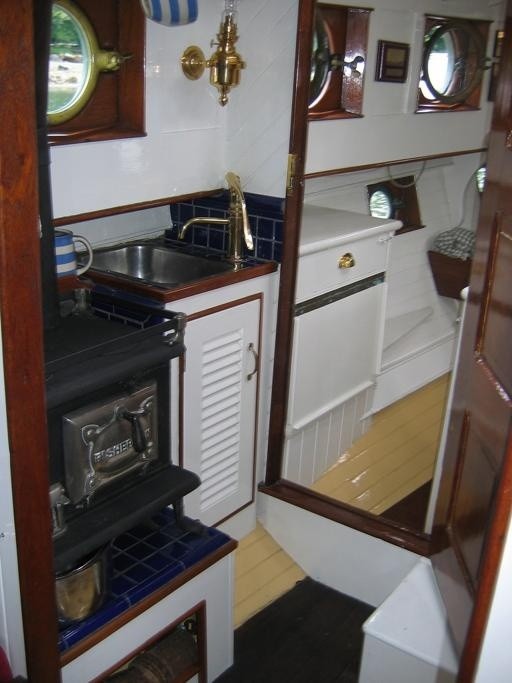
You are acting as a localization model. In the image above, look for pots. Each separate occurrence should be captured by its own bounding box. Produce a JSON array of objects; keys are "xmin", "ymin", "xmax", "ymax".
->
[{"xmin": 55, "ymin": 539, "xmax": 115, "ymax": 630}]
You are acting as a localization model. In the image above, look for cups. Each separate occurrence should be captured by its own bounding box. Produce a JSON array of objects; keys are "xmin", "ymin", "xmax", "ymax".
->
[{"xmin": 74, "ymin": 288, "xmax": 92, "ymax": 310}]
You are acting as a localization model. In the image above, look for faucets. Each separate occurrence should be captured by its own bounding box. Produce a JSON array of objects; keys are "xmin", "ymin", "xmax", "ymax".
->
[{"xmin": 177, "ymin": 173, "xmax": 253, "ymax": 260}]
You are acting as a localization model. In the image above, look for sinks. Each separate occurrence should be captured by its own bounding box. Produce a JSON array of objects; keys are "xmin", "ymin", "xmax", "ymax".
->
[{"xmin": 75, "ymin": 239, "xmax": 229, "ymax": 292}]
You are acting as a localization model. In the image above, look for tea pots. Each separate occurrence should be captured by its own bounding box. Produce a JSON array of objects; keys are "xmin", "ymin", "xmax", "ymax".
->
[{"xmin": 54, "ymin": 227, "xmax": 94, "ymax": 279}]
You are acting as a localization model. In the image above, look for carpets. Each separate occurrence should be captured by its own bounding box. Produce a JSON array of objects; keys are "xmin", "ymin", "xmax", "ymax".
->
[{"xmin": 214, "ymin": 575, "xmax": 380, "ymax": 683}]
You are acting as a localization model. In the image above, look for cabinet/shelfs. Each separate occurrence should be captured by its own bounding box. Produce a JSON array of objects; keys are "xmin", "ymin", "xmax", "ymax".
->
[{"xmin": 163, "ymin": 272, "xmax": 278, "ymax": 528}]
